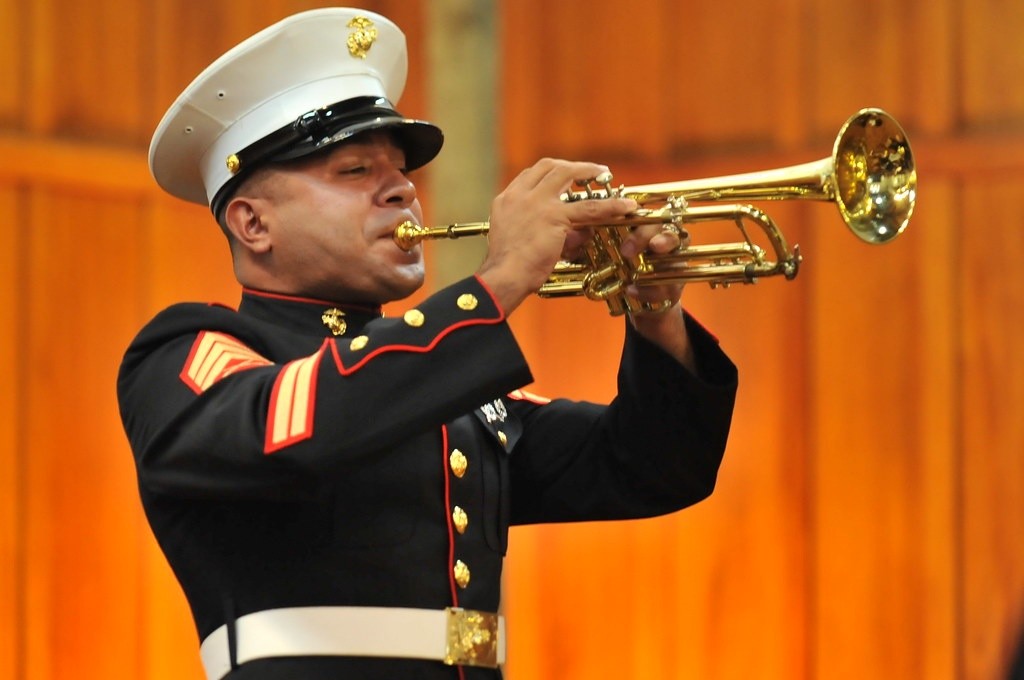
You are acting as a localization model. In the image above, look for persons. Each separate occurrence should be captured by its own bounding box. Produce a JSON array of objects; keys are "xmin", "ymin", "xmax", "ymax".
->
[{"xmin": 113, "ymin": 6, "xmax": 740, "ymax": 679}]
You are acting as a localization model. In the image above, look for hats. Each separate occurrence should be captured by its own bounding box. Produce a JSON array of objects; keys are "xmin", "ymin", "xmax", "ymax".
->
[{"xmin": 147, "ymin": 4, "xmax": 451, "ymax": 216}]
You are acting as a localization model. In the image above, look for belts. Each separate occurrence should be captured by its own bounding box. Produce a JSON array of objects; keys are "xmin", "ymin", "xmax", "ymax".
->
[{"xmin": 198, "ymin": 607, "xmax": 508, "ymax": 676}]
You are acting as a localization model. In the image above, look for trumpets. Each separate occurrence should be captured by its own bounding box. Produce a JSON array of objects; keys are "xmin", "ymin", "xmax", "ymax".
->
[{"xmin": 390, "ymin": 107, "xmax": 918, "ymax": 315}]
